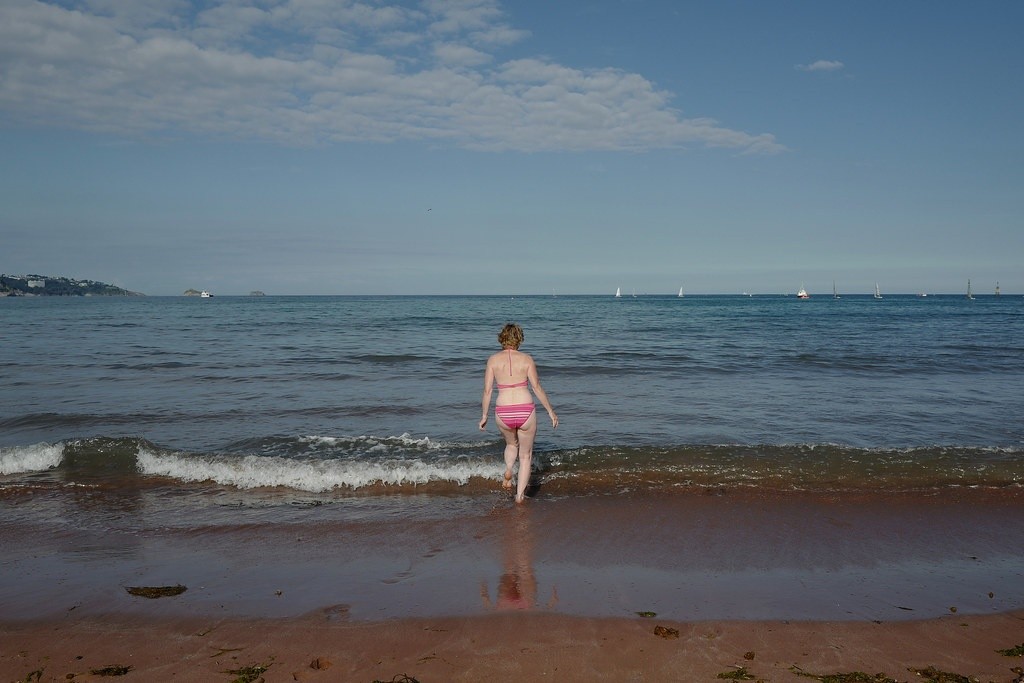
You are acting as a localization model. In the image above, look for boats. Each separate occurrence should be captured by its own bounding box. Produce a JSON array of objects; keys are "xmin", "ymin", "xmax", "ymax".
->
[{"xmin": 200, "ymin": 289, "xmax": 211, "ymax": 299}]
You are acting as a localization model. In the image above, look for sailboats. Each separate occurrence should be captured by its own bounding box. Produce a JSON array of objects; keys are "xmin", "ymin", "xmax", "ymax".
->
[
  {"xmin": 967, "ymin": 278, "xmax": 976, "ymax": 300},
  {"xmin": 614, "ymin": 288, "xmax": 623, "ymax": 297},
  {"xmin": 676, "ymin": 287, "xmax": 684, "ymax": 298},
  {"xmin": 798, "ymin": 281, "xmax": 810, "ymax": 299},
  {"xmin": 832, "ymin": 282, "xmax": 842, "ymax": 300},
  {"xmin": 873, "ymin": 282, "xmax": 883, "ymax": 299}
]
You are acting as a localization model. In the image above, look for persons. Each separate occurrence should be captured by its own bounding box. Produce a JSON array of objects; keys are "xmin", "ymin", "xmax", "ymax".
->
[{"xmin": 477, "ymin": 323, "xmax": 558, "ymax": 503}]
